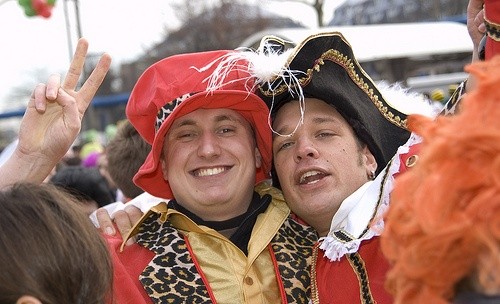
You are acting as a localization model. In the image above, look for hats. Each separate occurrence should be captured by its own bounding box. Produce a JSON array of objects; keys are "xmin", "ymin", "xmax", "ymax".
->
[
  {"xmin": 125, "ymin": 50, "xmax": 275, "ymax": 198},
  {"xmin": 254, "ymin": 32, "xmax": 420, "ymax": 173}
]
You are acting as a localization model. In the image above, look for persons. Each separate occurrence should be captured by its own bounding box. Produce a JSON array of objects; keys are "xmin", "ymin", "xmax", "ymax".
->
[
  {"xmin": 92, "ymin": 1, "xmax": 488, "ymax": 304},
  {"xmin": 0, "ymin": 83, "xmax": 457, "ymax": 227},
  {"xmin": 0, "ymin": 180, "xmax": 114, "ymax": 304},
  {"xmin": 0, "ymin": 38, "xmax": 316, "ymax": 304}
]
